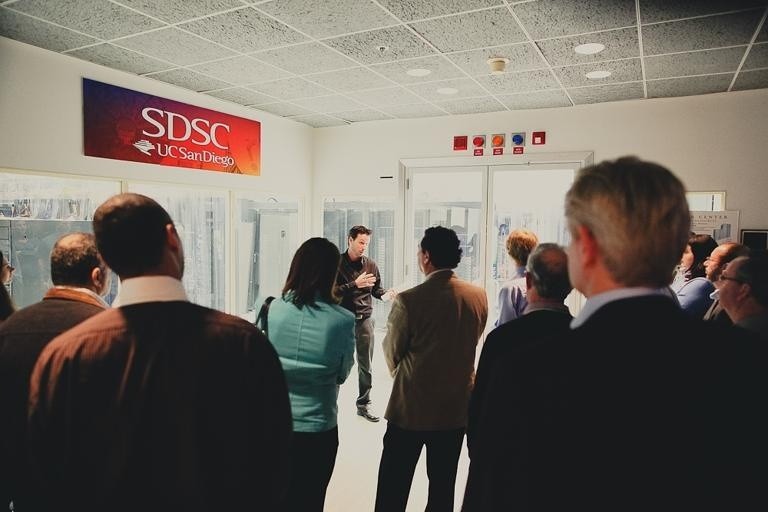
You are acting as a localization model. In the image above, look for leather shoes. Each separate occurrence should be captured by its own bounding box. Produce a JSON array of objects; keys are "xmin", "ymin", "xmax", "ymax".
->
[{"xmin": 356, "ymin": 408, "xmax": 380, "ymax": 423}]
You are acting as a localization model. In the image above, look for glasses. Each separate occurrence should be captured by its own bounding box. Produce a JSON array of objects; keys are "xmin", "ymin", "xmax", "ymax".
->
[
  {"xmin": 706, "ymin": 257, "xmax": 724, "ymax": 265},
  {"xmin": 719, "ymin": 274, "xmax": 745, "ymax": 285}
]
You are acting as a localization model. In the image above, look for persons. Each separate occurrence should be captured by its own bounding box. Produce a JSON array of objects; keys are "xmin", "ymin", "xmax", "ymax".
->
[
  {"xmin": 376, "ymin": 226, "xmax": 574, "ymax": 512},
  {"xmin": 256, "ymin": 236, "xmax": 356, "ymax": 512},
  {"xmin": 455, "ymin": 154, "xmax": 768, "ymax": 510},
  {"xmin": 334, "ymin": 225, "xmax": 397, "ymax": 422},
  {"xmin": 671, "ymin": 230, "xmax": 767, "ymax": 330},
  {"xmin": 22, "ymin": 193, "xmax": 292, "ymax": 511},
  {"xmin": 0, "ymin": 231, "xmax": 111, "ymax": 512}
]
